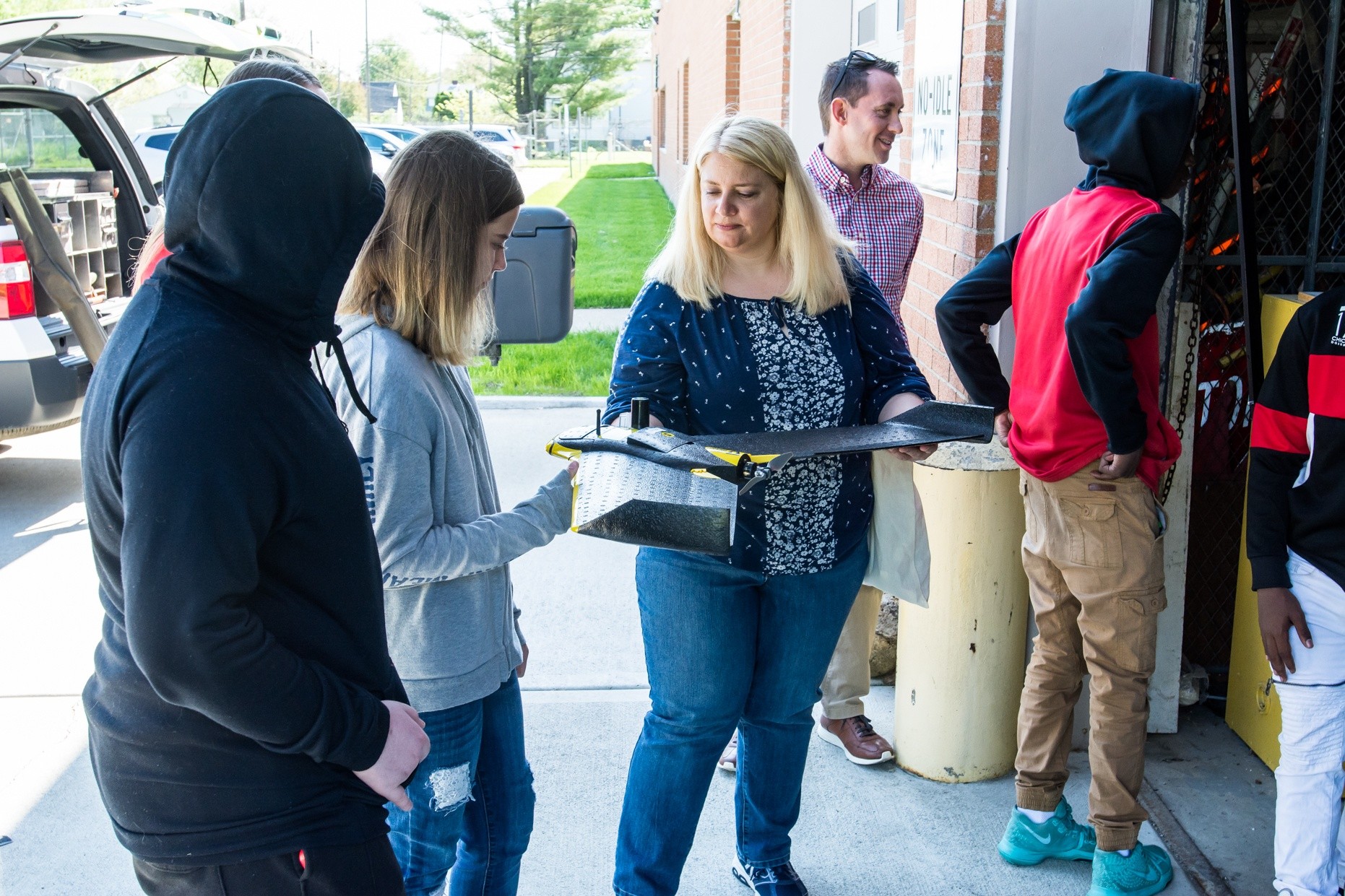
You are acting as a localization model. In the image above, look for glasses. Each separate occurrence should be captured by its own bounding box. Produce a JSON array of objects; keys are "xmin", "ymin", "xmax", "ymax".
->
[{"xmin": 830, "ymin": 50, "xmax": 893, "ymax": 102}]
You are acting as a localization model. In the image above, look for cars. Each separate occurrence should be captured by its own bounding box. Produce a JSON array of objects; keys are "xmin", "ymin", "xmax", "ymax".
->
[{"xmin": 130, "ymin": 121, "xmax": 526, "ymax": 201}]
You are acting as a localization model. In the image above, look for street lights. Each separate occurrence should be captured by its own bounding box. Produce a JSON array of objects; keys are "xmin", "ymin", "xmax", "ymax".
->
[{"xmin": 453, "ymin": 79, "xmax": 473, "ymax": 132}]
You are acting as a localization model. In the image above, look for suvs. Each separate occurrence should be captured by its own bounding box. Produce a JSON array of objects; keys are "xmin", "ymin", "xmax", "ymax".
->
[{"xmin": 0, "ymin": 2, "xmax": 315, "ymax": 429}]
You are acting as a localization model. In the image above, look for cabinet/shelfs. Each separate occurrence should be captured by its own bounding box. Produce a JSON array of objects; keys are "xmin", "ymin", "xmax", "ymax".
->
[{"xmin": 52, "ymin": 195, "xmax": 123, "ymax": 302}]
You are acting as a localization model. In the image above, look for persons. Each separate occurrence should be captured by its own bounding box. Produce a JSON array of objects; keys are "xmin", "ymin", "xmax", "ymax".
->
[
  {"xmin": 1244, "ymin": 292, "xmax": 1345, "ymax": 896},
  {"xmin": 308, "ymin": 131, "xmax": 580, "ymax": 896},
  {"xmin": 599, "ymin": 120, "xmax": 938, "ymax": 896},
  {"xmin": 935, "ymin": 68, "xmax": 1202, "ymax": 896},
  {"xmin": 716, "ymin": 50, "xmax": 924, "ymax": 771},
  {"xmin": 84, "ymin": 61, "xmax": 431, "ymax": 896}
]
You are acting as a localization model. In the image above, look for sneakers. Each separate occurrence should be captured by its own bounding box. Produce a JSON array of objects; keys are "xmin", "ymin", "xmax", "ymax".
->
[
  {"xmin": 998, "ymin": 794, "xmax": 1097, "ymax": 866},
  {"xmin": 1086, "ymin": 840, "xmax": 1174, "ymax": 896},
  {"xmin": 731, "ymin": 852, "xmax": 810, "ymax": 896},
  {"xmin": 717, "ymin": 731, "xmax": 738, "ymax": 772},
  {"xmin": 817, "ymin": 713, "xmax": 895, "ymax": 765}
]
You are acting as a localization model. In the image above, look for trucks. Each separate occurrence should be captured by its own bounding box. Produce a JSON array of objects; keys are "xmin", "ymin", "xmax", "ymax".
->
[{"xmin": 545, "ymin": 89, "xmax": 652, "ymax": 152}]
